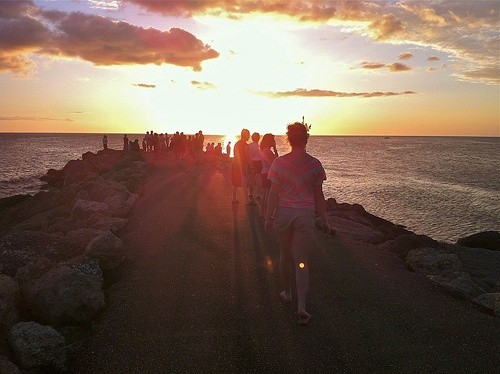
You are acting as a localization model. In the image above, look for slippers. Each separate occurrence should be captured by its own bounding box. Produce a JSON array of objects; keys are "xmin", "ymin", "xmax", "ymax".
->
[
  {"xmin": 280, "ymin": 291, "xmax": 292, "ymax": 304},
  {"xmin": 298, "ymin": 314, "xmax": 311, "ymax": 323}
]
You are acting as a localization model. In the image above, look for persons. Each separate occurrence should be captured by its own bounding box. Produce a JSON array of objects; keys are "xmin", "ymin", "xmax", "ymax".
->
[
  {"xmin": 103, "ymin": 135, "xmax": 108, "ymax": 148},
  {"xmin": 206, "ymin": 142, "xmax": 222, "ymax": 160},
  {"xmin": 232, "ymin": 130, "xmax": 278, "ymax": 217},
  {"xmin": 264, "ymin": 123, "xmax": 331, "ymax": 323},
  {"xmin": 226, "ymin": 142, "xmax": 231, "ymax": 156},
  {"xmin": 142, "ymin": 130, "xmax": 204, "ymax": 159},
  {"xmin": 124, "ymin": 134, "xmax": 144, "ymax": 161}
]
